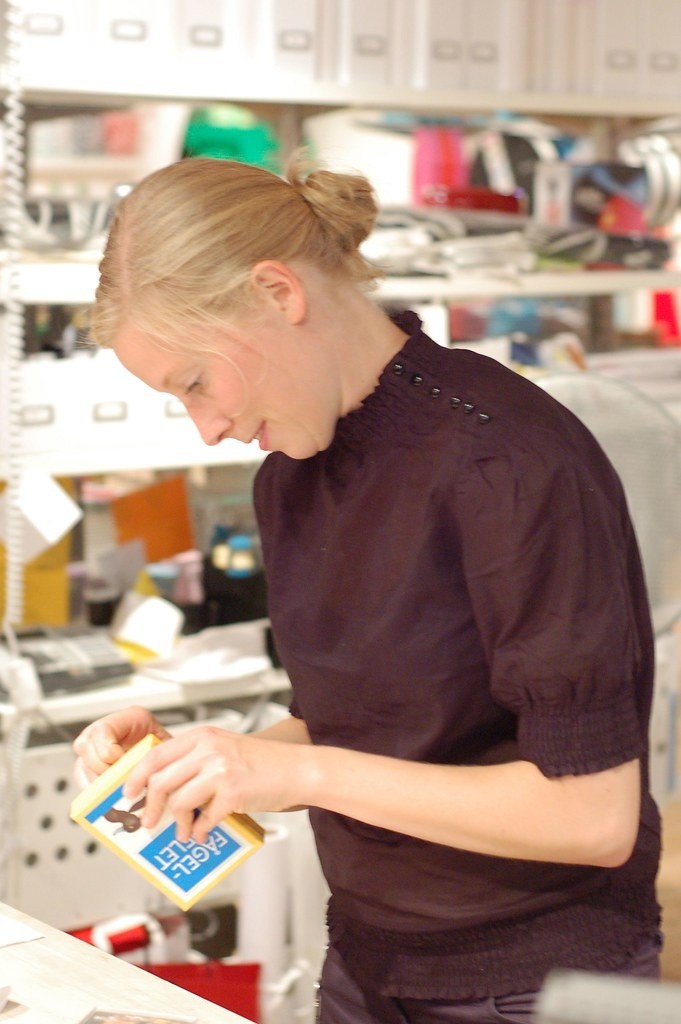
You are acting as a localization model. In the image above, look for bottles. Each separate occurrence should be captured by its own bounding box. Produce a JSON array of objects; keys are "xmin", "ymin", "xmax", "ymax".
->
[
  {"xmin": 226, "ymin": 535, "xmax": 256, "ymax": 579},
  {"xmin": 210, "ymin": 523, "xmax": 241, "ymax": 571}
]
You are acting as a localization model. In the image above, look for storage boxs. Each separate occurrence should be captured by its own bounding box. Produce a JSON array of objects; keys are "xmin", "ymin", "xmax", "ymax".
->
[{"xmin": 69, "ymin": 731, "xmax": 265, "ymax": 912}]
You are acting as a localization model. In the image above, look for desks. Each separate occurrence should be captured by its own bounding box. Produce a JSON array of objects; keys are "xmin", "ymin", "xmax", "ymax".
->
[{"xmin": 0, "ymin": 900, "xmax": 260, "ymax": 1024}]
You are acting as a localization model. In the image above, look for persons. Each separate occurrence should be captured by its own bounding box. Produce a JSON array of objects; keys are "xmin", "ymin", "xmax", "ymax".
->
[{"xmin": 72, "ymin": 157, "xmax": 664, "ymax": 1024}]
